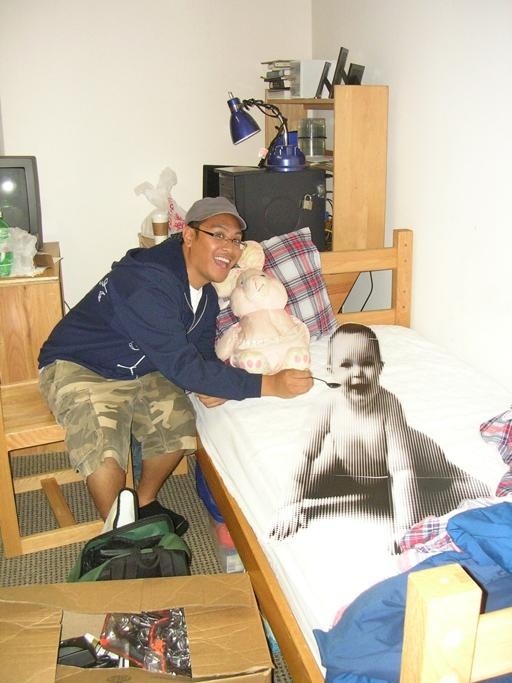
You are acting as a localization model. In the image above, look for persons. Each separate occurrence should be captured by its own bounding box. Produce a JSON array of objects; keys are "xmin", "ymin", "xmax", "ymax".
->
[
  {"xmin": 266, "ymin": 320, "xmax": 488, "ymax": 552},
  {"xmin": 37, "ymin": 194, "xmax": 314, "ymax": 539}
]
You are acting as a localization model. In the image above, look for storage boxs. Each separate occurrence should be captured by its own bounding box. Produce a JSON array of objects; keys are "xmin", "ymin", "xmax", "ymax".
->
[{"xmin": 1, "ymin": 573, "xmax": 276, "ymax": 683}]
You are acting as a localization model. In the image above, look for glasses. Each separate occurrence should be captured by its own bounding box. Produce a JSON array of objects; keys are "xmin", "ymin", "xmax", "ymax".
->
[{"xmin": 190, "ymin": 225, "xmax": 248, "ymax": 251}]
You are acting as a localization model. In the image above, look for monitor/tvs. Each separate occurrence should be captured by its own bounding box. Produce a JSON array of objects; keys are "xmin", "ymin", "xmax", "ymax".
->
[
  {"xmin": 203, "ymin": 165, "xmax": 239, "ymax": 199},
  {"xmin": 0, "ymin": 156, "xmax": 43, "ymax": 250}
]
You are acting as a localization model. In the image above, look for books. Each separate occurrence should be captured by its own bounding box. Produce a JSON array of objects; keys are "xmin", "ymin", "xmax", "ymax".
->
[{"xmin": 261, "ymin": 58, "xmax": 295, "ymax": 98}]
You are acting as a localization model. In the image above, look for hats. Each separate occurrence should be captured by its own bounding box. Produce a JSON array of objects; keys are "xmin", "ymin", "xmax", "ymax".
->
[{"xmin": 185, "ymin": 195, "xmax": 247, "ymax": 230}]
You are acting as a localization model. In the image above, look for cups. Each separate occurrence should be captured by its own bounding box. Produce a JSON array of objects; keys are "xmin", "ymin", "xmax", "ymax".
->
[{"xmin": 150, "ymin": 213, "xmax": 170, "ymax": 245}]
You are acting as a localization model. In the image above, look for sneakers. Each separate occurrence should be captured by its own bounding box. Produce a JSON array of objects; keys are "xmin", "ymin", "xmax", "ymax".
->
[{"xmin": 139, "ymin": 501, "xmax": 190, "ymax": 537}]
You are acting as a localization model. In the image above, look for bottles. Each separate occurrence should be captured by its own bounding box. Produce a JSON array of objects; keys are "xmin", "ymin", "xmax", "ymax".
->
[{"xmin": 0, "ymin": 210, "xmax": 13, "ymax": 276}]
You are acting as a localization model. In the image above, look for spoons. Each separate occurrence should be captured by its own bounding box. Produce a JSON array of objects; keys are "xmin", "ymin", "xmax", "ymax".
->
[{"xmin": 307, "ymin": 375, "xmax": 342, "ymax": 390}]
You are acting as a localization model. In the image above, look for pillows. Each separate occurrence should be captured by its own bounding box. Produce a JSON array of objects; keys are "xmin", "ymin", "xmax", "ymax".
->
[{"xmin": 216, "ymin": 226, "xmax": 340, "ymax": 365}]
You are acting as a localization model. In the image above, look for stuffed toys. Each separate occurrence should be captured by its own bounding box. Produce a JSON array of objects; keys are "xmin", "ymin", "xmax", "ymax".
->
[{"xmin": 213, "ymin": 239, "xmax": 311, "ymax": 376}]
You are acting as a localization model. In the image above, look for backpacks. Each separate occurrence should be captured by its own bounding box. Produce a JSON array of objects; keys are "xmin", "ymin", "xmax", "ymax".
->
[{"xmin": 66, "ymin": 485, "xmax": 192, "ymax": 581}]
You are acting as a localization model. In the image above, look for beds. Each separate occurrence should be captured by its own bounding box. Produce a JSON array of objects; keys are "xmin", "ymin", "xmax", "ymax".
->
[{"xmin": 195, "ymin": 228, "xmax": 511, "ymax": 683}]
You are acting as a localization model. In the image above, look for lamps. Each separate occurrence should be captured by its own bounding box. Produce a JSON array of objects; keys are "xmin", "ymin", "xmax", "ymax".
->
[{"xmin": 226, "ymin": 90, "xmax": 307, "ymax": 172}]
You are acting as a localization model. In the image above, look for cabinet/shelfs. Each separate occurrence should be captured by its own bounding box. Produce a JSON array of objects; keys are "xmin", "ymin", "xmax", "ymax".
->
[{"xmin": 265, "ymin": 83, "xmax": 388, "ymax": 250}]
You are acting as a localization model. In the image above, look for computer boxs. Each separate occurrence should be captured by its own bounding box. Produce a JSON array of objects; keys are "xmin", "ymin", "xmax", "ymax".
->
[{"xmin": 217, "ymin": 166, "xmax": 327, "ymax": 252}]
